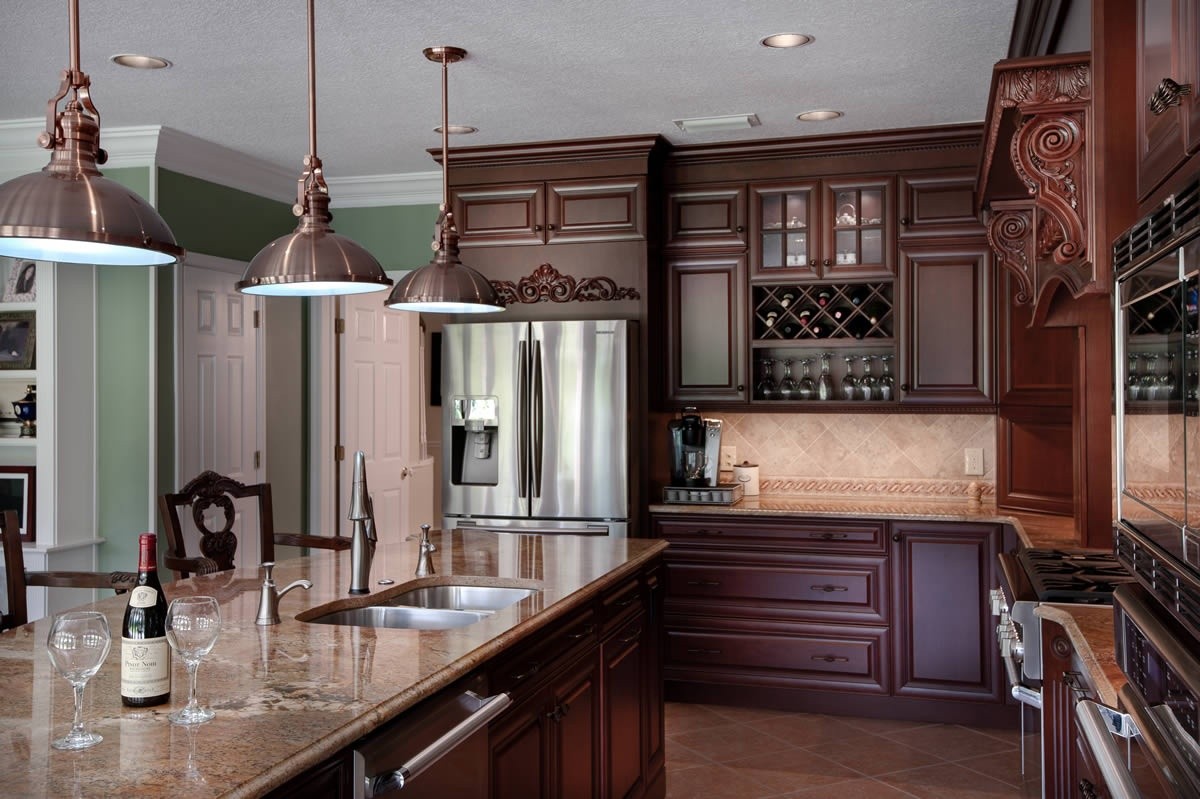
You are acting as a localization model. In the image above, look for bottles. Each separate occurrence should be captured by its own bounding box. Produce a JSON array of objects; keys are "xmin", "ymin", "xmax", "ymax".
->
[
  {"xmin": 120, "ymin": 533, "xmax": 172, "ymax": 708},
  {"xmin": 765, "ymin": 287, "xmax": 887, "ymax": 341}
]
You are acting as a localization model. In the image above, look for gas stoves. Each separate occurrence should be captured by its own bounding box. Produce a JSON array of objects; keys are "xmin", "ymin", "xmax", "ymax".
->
[{"xmin": 1015, "ymin": 546, "xmax": 1137, "ymax": 605}]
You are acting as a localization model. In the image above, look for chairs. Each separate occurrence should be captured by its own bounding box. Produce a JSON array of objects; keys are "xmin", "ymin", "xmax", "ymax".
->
[
  {"xmin": 0, "ymin": 506, "xmax": 138, "ymax": 634},
  {"xmin": 162, "ymin": 469, "xmax": 274, "ymax": 576}
]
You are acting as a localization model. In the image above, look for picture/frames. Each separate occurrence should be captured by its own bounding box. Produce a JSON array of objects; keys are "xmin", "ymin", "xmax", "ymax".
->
[{"xmin": 0, "ymin": 465, "xmax": 35, "ymax": 542}]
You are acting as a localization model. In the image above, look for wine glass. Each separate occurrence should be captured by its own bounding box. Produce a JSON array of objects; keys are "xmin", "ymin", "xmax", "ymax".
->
[
  {"xmin": 1126, "ymin": 351, "xmax": 1178, "ymax": 400},
  {"xmin": 165, "ymin": 595, "xmax": 222, "ymax": 724},
  {"xmin": 754, "ymin": 351, "xmax": 896, "ymax": 402},
  {"xmin": 46, "ymin": 611, "xmax": 112, "ymax": 750}
]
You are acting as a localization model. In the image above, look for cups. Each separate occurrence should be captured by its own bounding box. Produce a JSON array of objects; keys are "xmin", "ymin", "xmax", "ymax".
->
[
  {"xmin": 683, "ymin": 450, "xmax": 709, "ymax": 479},
  {"xmin": 733, "ymin": 460, "xmax": 761, "ymax": 495}
]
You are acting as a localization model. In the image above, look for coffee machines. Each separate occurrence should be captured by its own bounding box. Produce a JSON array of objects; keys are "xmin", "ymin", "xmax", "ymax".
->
[{"xmin": 667, "ymin": 407, "xmax": 712, "ymax": 488}]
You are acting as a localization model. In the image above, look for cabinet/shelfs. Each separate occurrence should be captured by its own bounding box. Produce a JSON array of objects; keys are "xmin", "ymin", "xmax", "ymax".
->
[{"xmin": 0, "ymin": 0, "xmax": 1200, "ymax": 799}]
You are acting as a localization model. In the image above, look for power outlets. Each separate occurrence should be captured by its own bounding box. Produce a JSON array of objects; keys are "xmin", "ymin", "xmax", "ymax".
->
[{"xmin": 965, "ymin": 448, "xmax": 984, "ymax": 476}]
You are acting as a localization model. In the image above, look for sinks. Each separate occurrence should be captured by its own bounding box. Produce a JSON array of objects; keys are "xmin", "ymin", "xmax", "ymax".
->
[
  {"xmin": 293, "ymin": 597, "xmax": 496, "ymax": 631},
  {"xmin": 370, "ymin": 573, "xmax": 545, "ymax": 614}
]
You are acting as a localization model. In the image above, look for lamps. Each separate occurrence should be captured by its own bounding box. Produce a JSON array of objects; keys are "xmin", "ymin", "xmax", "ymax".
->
[
  {"xmin": 384, "ymin": 46, "xmax": 508, "ymax": 315},
  {"xmin": 0, "ymin": 0, "xmax": 182, "ymax": 266},
  {"xmin": 236, "ymin": 0, "xmax": 395, "ymax": 297}
]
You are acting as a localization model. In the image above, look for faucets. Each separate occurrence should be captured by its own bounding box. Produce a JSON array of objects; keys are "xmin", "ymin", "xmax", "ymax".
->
[
  {"xmin": 415, "ymin": 524, "xmax": 438, "ymax": 575},
  {"xmin": 255, "ymin": 560, "xmax": 314, "ymax": 626},
  {"xmin": 348, "ymin": 450, "xmax": 377, "ymax": 595}
]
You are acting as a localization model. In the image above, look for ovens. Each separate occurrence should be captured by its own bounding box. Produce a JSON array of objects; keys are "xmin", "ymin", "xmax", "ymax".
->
[{"xmin": 987, "ymin": 574, "xmax": 1043, "ymax": 799}]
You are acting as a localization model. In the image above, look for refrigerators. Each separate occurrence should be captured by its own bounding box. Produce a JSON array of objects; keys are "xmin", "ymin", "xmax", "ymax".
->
[{"xmin": 438, "ymin": 319, "xmax": 641, "ymax": 540}]
